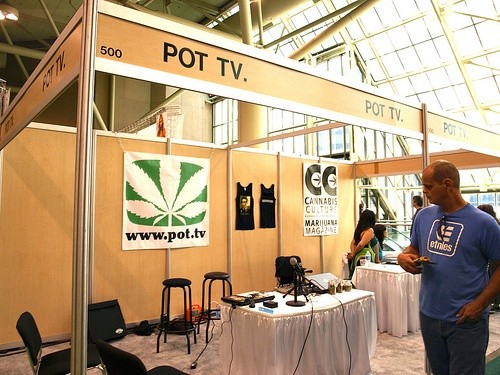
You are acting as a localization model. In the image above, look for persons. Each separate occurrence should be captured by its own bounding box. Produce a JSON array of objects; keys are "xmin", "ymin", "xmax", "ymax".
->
[
  {"xmin": 477, "ymin": 204, "xmax": 500, "ymax": 225},
  {"xmin": 346, "ymin": 210, "xmax": 375, "ymax": 279},
  {"xmin": 410, "ymin": 196, "xmax": 424, "ymax": 238},
  {"xmin": 397, "ymin": 160, "xmax": 500, "ymax": 375},
  {"xmin": 370, "ymin": 224, "xmax": 388, "ymax": 262}
]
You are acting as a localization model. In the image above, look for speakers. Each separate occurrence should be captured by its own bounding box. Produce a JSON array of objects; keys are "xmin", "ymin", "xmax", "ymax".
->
[{"xmin": 88, "ymin": 299, "xmax": 127, "ymax": 342}]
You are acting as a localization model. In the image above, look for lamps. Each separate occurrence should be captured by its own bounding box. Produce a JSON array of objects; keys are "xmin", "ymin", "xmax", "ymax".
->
[{"xmin": 0, "ymin": 3, "xmax": 20, "ymax": 22}]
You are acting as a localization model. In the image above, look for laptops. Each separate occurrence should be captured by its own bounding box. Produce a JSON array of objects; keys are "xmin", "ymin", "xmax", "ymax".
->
[{"xmin": 221, "ymin": 291, "xmax": 275, "ymax": 306}]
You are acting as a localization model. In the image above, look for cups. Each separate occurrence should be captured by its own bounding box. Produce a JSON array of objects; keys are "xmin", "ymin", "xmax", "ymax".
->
[{"xmin": 327, "ymin": 279, "xmax": 353, "ymax": 294}]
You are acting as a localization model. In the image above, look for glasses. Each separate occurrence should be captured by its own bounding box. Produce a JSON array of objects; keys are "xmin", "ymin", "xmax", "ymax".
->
[{"xmin": 437, "ymin": 216, "xmax": 446, "ymax": 241}]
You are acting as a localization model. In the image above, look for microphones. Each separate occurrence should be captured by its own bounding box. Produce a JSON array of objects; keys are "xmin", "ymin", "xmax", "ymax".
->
[{"xmin": 290, "ymin": 258, "xmax": 303, "ymax": 277}]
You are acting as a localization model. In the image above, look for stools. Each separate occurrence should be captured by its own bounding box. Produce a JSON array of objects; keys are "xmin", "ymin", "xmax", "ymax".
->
[
  {"xmin": 197, "ymin": 271, "xmax": 232, "ymax": 343},
  {"xmin": 157, "ymin": 277, "xmax": 197, "ymax": 353}
]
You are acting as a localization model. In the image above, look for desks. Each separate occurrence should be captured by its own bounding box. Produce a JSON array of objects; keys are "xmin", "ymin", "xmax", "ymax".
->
[
  {"xmin": 221, "ymin": 289, "xmax": 378, "ymax": 375},
  {"xmin": 353, "ymin": 262, "xmax": 420, "ymax": 337}
]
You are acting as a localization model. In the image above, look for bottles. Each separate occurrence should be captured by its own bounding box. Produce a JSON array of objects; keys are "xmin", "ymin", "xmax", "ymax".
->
[{"xmin": 365, "ymin": 251, "xmax": 371, "ymax": 267}]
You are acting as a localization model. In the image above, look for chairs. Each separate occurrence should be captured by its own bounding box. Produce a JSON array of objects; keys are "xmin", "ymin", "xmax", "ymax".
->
[
  {"xmin": 15, "ymin": 311, "xmax": 106, "ymax": 375},
  {"xmin": 95, "ymin": 339, "xmax": 190, "ymax": 375}
]
